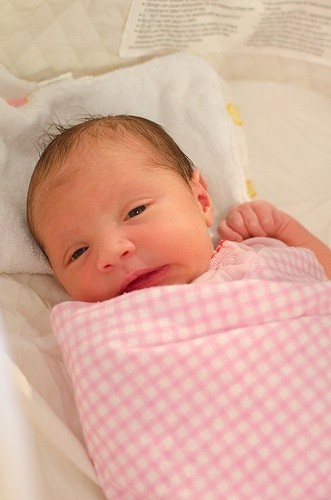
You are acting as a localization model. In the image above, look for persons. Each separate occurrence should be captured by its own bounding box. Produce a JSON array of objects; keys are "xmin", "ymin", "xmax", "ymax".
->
[{"xmin": 25, "ymin": 107, "xmax": 331, "ymax": 500}]
